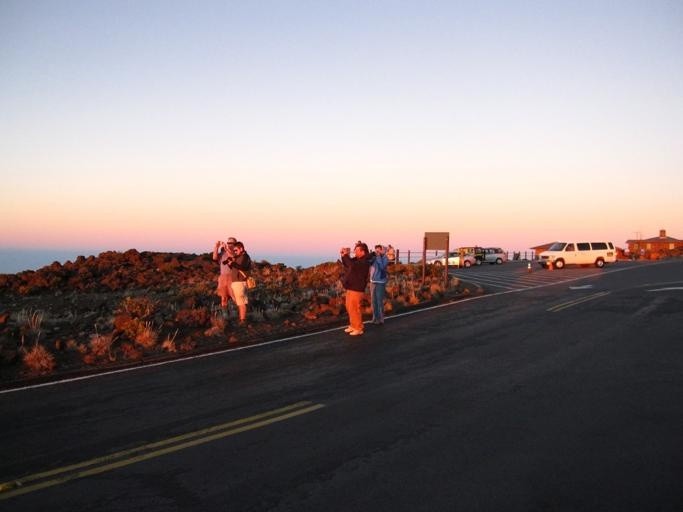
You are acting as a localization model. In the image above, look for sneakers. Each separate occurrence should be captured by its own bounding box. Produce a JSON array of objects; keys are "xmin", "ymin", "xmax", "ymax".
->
[
  {"xmin": 230, "ymin": 318, "xmax": 248, "ymax": 328},
  {"xmin": 343, "ymin": 324, "xmax": 365, "ymax": 336}
]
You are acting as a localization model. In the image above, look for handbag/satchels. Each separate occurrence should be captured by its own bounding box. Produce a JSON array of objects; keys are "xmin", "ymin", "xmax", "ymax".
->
[{"xmin": 246, "ymin": 275, "xmax": 256, "ymax": 289}]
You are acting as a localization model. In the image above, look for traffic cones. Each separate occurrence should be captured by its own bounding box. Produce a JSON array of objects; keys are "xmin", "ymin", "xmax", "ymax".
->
[{"xmin": 548, "ymin": 261, "xmax": 554, "ymax": 271}]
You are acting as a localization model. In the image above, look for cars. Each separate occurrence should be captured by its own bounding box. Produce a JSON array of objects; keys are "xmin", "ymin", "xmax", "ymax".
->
[{"xmin": 425, "ymin": 247, "xmax": 508, "ymax": 269}]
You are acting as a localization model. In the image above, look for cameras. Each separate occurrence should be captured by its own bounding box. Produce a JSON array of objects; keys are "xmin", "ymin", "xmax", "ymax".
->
[
  {"xmin": 219, "ymin": 241, "xmax": 223, "ymax": 245},
  {"xmin": 222, "ymin": 256, "xmax": 232, "ymax": 265}
]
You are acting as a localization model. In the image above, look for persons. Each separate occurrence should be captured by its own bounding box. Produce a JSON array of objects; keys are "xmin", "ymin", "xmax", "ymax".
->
[
  {"xmin": 385, "ymin": 245, "xmax": 395, "ymax": 265},
  {"xmin": 227, "ymin": 242, "xmax": 255, "ymax": 326},
  {"xmin": 339, "ymin": 243, "xmax": 372, "ymax": 336},
  {"xmin": 367, "ymin": 245, "xmax": 387, "ymax": 326},
  {"xmin": 212, "ymin": 236, "xmax": 238, "ymax": 319}
]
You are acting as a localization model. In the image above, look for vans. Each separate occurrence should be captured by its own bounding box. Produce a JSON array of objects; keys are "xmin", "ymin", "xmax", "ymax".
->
[{"xmin": 537, "ymin": 241, "xmax": 617, "ymax": 269}]
